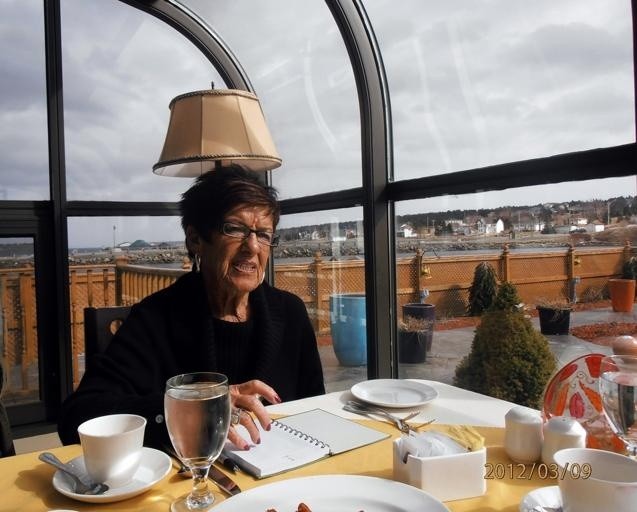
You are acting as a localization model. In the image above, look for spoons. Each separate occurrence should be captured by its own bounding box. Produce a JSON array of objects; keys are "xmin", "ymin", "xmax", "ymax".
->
[
  {"xmin": 39, "ymin": 451, "xmax": 109, "ymax": 495},
  {"xmin": 168, "ymin": 448, "xmax": 191, "ymax": 478}
]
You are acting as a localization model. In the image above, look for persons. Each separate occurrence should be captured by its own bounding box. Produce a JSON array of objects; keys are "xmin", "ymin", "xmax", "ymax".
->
[{"xmin": 57, "ymin": 163, "xmax": 325, "ymax": 448}]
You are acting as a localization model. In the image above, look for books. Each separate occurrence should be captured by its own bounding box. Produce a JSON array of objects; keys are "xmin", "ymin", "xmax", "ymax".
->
[{"xmin": 220, "ymin": 407, "xmax": 393, "ymax": 480}]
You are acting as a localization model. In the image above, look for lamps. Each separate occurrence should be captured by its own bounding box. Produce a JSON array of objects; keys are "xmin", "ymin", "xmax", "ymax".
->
[{"xmin": 152, "ymin": 82, "xmax": 283, "ymax": 177}]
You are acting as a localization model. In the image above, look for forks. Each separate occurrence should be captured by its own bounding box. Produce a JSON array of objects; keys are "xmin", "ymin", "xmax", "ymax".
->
[{"xmin": 343, "ymin": 400, "xmax": 436, "ymax": 432}]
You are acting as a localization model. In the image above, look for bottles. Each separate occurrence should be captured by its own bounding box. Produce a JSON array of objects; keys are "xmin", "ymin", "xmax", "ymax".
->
[{"xmin": 504, "ymin": 407, "xmax": 588, "ymax": 473}]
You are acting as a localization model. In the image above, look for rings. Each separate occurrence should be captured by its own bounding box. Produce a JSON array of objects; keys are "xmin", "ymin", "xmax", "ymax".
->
[{"xmin": 228, "ymin": 409, "xmax": 245, "ymax": 428}]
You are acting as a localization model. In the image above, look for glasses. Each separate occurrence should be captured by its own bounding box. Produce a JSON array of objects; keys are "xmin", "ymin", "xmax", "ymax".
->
[{"xmin": 213, "ymin": 223, "xmax": 280, "ymax": 247}]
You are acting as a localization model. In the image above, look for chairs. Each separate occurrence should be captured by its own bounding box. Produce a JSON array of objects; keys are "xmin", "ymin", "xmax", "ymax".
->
[{"xmin": 84, "ymin": 306, "xmax": 130, "ymax": 370}]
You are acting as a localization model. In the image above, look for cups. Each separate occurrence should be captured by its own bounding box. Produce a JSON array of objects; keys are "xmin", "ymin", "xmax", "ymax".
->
[
  {"xmin": 76, "ymin": 413, "xmax": 147, "ymax": 488},
  {"xmin": 553, "ymin": 448, "xmax": 637, "ymax": 512}
]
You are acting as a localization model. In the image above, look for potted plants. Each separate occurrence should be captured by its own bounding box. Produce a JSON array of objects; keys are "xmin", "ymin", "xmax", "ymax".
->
[
  {"xmin": 536, "ymin": 297, "xmax": 572, "ymax": 336},
  {"xmin": 397, "ymin": 315, "xmax": 430, "ymax": 362}
]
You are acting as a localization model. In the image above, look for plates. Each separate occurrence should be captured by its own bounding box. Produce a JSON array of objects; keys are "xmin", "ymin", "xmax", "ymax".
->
[
  {"xmin": 520, "ymin": 484, "xmax": 564, "ymax": 511},
  {"xmin": 206, "ymin": 474, "xmax": 450, "ymax": 512},
  {"xmin": 350, "ymin": 378, "xmax": 438, "ymax": 408},
  {"xmin": 51, "ymin": 446, "xmax": 173, "ymax": 504}
]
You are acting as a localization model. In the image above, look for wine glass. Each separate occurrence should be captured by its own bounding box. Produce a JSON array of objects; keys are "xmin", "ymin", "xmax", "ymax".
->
[
  {"xmin": 163, "ymin": 372, "xmax": 232, "ymax": 512},
  {"xmin": 597, "ymin": 355, "xmax": 637, "ymax": 458}
]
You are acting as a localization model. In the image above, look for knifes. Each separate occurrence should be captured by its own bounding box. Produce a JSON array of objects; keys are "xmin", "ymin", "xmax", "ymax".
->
[{"xmin": 209, "ymin": 465, "xmax": 242, "ymax": 495}]
